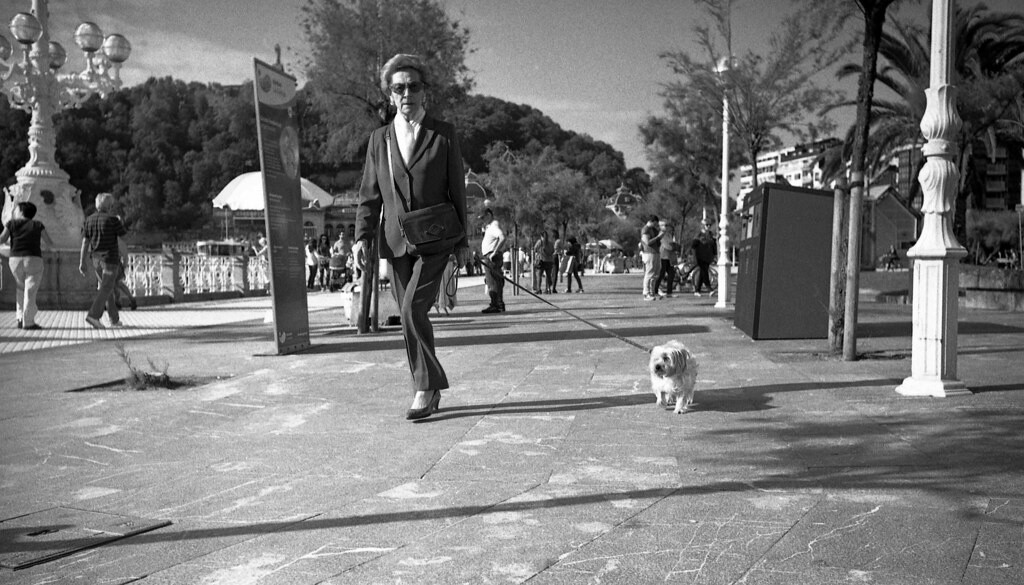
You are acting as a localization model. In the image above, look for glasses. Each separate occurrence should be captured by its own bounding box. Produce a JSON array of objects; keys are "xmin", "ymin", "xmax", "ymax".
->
[
  {"xmin": 704, "ymin": 224, "xmax": 712, "ymax": 227},
  {"xmin": 390, "ymin": 82, "xmax": 424, "ymax": 95}
]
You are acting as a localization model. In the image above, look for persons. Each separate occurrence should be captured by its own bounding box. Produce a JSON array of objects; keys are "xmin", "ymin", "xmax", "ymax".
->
[
  {"xmin": 639, "ymin": 215, "xmax": 718, "ymax": 301},
  {"xmin": 79, "ymin": 192, "xmax": 137, "ymax": 328},
  {"xmin": 0, "ymin": 202, "xmax": 59, "ymax": 330},
  {"xmin": 351, "ymin": 53, "xmax": 470, "ymax": 420},
  {"xmin": 251, "ymin": 230, "xmax": 356, "ymax": 292},
  {"xmin": 435, "ymin": 209, "xmax": 615, "ymax": 316}
]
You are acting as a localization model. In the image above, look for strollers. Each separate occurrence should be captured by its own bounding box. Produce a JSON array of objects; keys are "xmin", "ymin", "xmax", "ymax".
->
[{"xmin": 327, "ymin": 254, "xmax": 350, "ymax": 289}]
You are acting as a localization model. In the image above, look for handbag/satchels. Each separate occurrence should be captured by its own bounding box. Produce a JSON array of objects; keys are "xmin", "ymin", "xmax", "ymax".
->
[
  {"xmin": 560, "ymin": 255, "xmax": 576, "ymax": 273},
  {"xmin": 399, "ymin": 202, "xmax": 466, "ymax": 255}
]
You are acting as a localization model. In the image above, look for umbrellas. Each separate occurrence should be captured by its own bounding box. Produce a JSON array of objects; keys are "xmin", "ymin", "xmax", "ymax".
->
[{"xmin": 211, "ymin": 171, "xmax": 334, "ymax": 231}]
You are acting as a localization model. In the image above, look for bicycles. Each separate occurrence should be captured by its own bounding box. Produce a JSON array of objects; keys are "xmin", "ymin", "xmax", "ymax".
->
[
  {"xmin": 878, "ymin": 257, "xmax": 902, "ymax": 273},
  {"xmin": 656, "ymin": 257, "xmax": 719, "ymax": 293}
]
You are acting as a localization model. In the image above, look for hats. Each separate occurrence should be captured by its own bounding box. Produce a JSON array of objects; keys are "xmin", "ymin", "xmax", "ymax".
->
[{"xmin": 477, "ymin": 207, "xmax": 490, "ymax": 219}]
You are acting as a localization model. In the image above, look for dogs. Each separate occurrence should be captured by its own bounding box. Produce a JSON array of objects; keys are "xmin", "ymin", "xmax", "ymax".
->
[{"xmin": 647, "ymin": 339, "xmax": 698, "ymax": 414}]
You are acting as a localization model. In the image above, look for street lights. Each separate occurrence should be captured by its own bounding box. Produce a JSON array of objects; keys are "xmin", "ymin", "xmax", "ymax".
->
[
  {"xmin": 0, "ymin": 11, "xmax": 133, "ymax": 247},
  {"xmin": 712, "ymin": 55, "xmax": 738, "ymax": 309}
]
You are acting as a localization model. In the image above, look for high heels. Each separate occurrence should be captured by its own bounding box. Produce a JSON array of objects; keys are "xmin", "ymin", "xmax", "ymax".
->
[{"xmin": 405, "ymin": 390, "xmax": 441, "ymax": 420}]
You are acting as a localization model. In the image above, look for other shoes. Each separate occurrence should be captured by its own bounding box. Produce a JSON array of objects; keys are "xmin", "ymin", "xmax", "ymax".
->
[
  {"xmin": 24, "ymin": 324, "xmax": 42, "ymax": 330},
  {"xmin": 644, "ymin": 294, "xmax": 662, "ymax": 301},
  {"xmin": 693, "ymin": 292, "xmax": 702, "ymax": 297},
  {"xmin": 18, "ymin": 320, "xmax": 22, "ymax": 328},
  {"xmin": 710, "ymin": 290, "xmax": 715, "ymax": 298},
  {"xmin": 111, "ymin": 321, "xmax": 122, "ymax": 328},
  {"xmin": 565, "ymin": 289, "xmax": 571, "ymax": 293},
  {"xmin": 482, "ymin": 304, "xmax": 505, "ymax": 313},
  {"xmin": 535, "ymin": 290, "xmax": 542, "ymax": 294},
  {"xmin": 667, "ymin": 294, "xmax": 678, "ymax": 298},
  {"xmin": 546, "ymin": 290, "xmax": 551, "ymax": 294},
  {"xmin": 131, "ymin": 299, "xmax": 137, "ymax": 310},
  {"xmin": 576, "ymin": 289, "xmax": 584, "ymax": 293},
  {"xmin": 553, "ymin": 289, "xmax": 557, "ymax": 293},
  {"xmin": 85, "ymin": 316, "xmax": 105, "ymax": 329}
]
